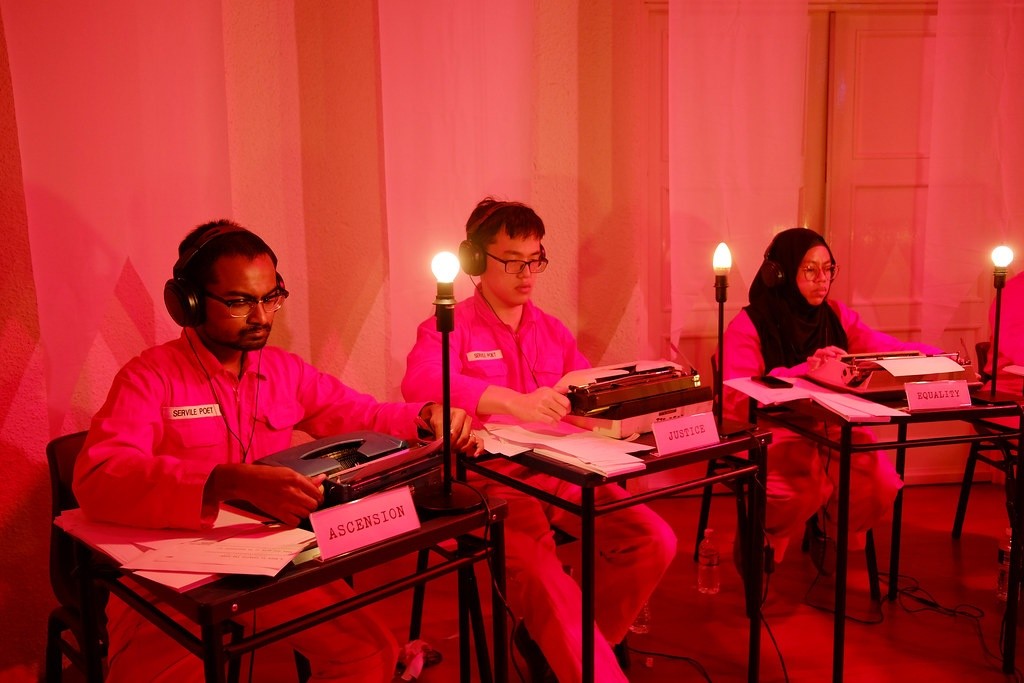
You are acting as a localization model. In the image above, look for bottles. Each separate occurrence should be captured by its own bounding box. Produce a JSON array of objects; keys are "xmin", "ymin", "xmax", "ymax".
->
[
  {"xmin": 996, "ymin": 527, "xmax": 1012, "ymax": 602},
  {"xmin": 629, "ymin": 601, "xmax": 649, "ymax": 634},
  {"xmin": 698, "ymin": 529, "xmax": 719, "ymax": 594}
]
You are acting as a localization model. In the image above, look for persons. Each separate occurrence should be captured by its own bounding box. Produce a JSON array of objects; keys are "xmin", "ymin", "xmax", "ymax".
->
[
  {"xmin": 401, "ymin": 198, "xmax": 679, "ymax": 683},
  {"xmin": 71, "ymin": 220, "xmax": 486, "ymax": 683},
  {"xmin": 710, "ymin": 228, "xmax": 980, "ymax": 624}
]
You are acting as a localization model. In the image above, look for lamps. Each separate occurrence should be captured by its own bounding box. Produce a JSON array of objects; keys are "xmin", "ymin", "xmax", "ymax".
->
[
  {"xmin": 971, "ymin": 244, "xmax": 1021, "ymax": 406},
  {"xmin": 413, "ymin": 249, "xmax": 488, "ymax": 523},
  {"xmin": 712, "ymin": 241, "xmax": 758, "ymax": 440}
]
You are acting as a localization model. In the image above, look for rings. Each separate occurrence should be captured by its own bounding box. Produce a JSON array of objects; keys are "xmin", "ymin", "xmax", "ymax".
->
[{"xmin": 469, "ymin": 433, "xmax": 476, "ymax": 438}]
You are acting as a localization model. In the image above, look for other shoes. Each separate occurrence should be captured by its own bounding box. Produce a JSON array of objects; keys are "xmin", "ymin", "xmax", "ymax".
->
[
  {"xmin": 732, "ymin": 522, "xmax": 747, "ymax": 575},
  {"xmin": 513, "ymin": 619, "xmax": 558, "ymax": 683},
  {"xmin": 805, "ymin": 513, "xmax": 836, "ymax": 578}
]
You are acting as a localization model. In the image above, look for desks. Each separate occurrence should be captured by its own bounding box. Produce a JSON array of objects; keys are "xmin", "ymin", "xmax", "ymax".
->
[
  {"xmin": 53, "ymin": 484, "xmax": 511, "ymax": 683},
  {"xmin": 453, "ymin": 417, "xmax": 773, "ymax": 683},
  {"xmin": 747, "ymin": 393, "xmax": 1024, "ymax": 683}
]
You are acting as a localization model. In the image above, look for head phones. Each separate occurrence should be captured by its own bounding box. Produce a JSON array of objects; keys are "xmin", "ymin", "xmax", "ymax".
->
[
  {"xmin": 164, "ymin": 226, "xmax": 285, "ymax": 329},
  {"xmin": 762, "ymin": 238, "xmax": 785, "ymax": 290},
  {"xmin": 458, "ymin": 203, "xmax": 542, "ymax": 282}
]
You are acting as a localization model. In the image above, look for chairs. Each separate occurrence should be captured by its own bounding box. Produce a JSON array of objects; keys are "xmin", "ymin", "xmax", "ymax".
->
[
  {"xmin": 408, "ymin": 429, "xmax": 633, "ymax": 683},
  {"xmin": 950, "ymin": 341, "xmax": 1024, "ymax": 542},
  {"xmin": 45, "ymin": 430, "xmax": 313, "ymax": 683},
  {"xmin": 692, "ymin": 353, "xmax": 884, "ymax": 619}
]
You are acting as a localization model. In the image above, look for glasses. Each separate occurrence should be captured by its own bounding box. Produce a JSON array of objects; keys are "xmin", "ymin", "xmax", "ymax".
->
[
  {"xmin": 482, "ymin": 250, "xmax": 549, "ymax": 274},
  {"xmin": 202, "ymin": 280, "xmax": 290, "ymax": 318},
  {"xmin": 799, "ymin": 264, "xmax": 841, "ymax": 282}
]
